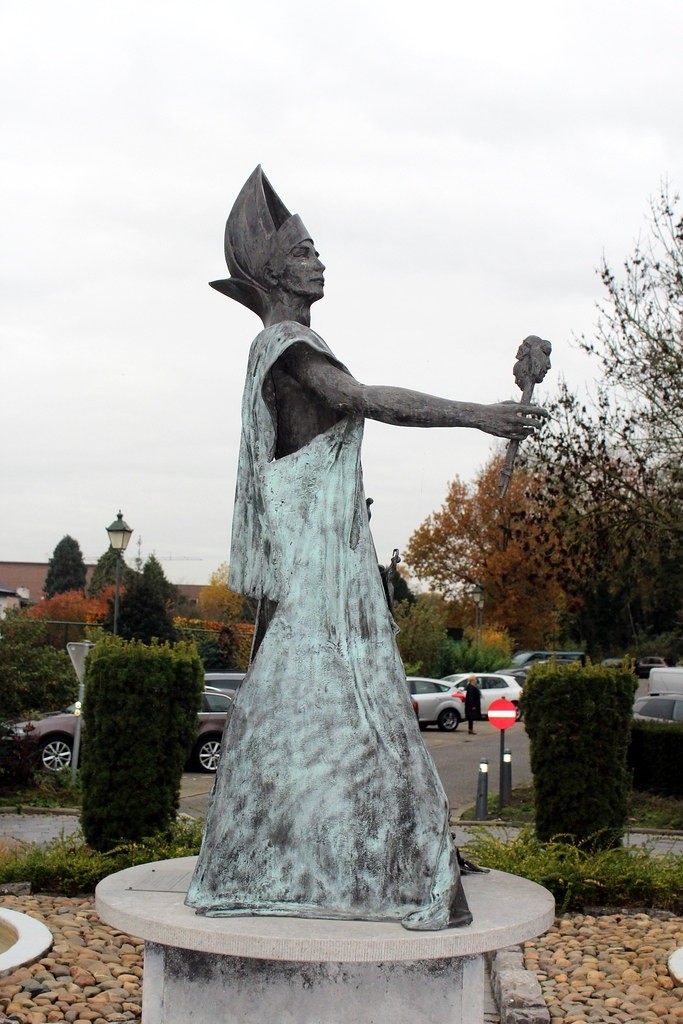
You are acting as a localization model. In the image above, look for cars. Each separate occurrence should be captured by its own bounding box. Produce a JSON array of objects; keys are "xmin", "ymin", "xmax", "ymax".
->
[
  {"xmin": 405, "ymin": 677, "xmax": 467, "ymax": 732},
  {"xmin": 631, "ymin": 692, "xmax": 683, "ymax": 727},
  {"xmin": 437, "ymin": 672, "xmax": 523, "ymax": 718},
  {"xmin": 0, "ymin": 685, "xmax": 232, "ymax": 774},
  {"xmin": 637, "ymin": 656, "xmax": 669, "ymax": 678},
  {"xmin": 496, "ymin": 668, "xmax": 527, "ymax": 686},
  {"xmin": 204, "ymin": 671, "xmax": 247, "ymax": 690},
  {"xmin": 66, "ymin": 686, "xmax": 238, "ymax": 718},
  {"xmin": 520, "ymin": 659, "xmax": 582, "ymax": 677},
  {"xmin": 600, "ymin": 658, "xmax": 626, "ymax": 668}
]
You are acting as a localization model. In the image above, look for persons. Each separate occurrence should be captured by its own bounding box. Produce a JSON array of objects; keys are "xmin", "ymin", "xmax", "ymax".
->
[
  {"xmin": 183, "ymin": 163, "xmax": 548, "ymax": 932},
  {"xmin": 464, "ymin": 677, "xmax": 484, "ymax": 735}
]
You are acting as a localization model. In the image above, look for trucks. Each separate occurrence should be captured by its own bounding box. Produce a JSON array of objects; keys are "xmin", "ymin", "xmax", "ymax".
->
[
  {"xmin": 648, "ymin": 666, "xmax": 683, "ymax": 695},
  {"xmin": 511, "ymin": 649, "xmax": 587, "ymax": 672}
]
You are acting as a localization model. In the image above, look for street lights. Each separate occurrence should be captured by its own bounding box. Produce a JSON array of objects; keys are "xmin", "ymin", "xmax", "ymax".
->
[{"xmin": 105, "ymin": 509, "xmax": 135, "ymax": 639}]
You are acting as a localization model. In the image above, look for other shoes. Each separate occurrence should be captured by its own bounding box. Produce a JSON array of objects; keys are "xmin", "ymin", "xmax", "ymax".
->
[{"xmin": 469, "ymin": 729, "xmax": 477, "ymax": 735}]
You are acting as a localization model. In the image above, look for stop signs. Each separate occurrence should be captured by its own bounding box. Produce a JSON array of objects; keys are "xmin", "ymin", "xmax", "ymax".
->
[{"xmin": 488, "ymin": 699, "xmax": 518, "ymax": 730}]
